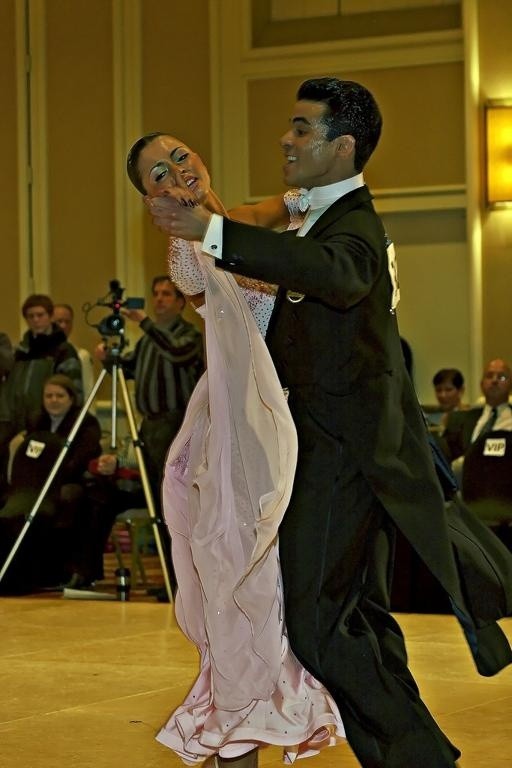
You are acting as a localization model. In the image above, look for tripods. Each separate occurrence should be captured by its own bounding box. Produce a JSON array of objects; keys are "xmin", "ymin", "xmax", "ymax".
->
[{"xmin": 0, "ymin": 353, "xmax": 173, "ymax": 603}]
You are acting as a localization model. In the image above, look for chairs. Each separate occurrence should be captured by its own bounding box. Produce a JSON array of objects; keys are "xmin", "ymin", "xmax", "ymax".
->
[{"xmin": 110, "ymin": 508, "xmax": 151, "ymax": 591}]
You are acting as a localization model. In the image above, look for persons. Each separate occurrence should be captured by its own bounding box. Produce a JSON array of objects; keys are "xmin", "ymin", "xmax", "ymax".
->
[
  {"xmin": 94, "ymin": 276, "xmax": 205, "ymax": 603},
  {"xmin": 127, "ymin": 129, "xmax": 350, "ymax": 766},
  {"xmin": 142, "ymin": 75, "xmax": 512, "ymax": 765},
  {"xmin": 1, "ymin": 294, "xmax": 121, "ymax": 598},
  {"xmin": 429, "ymin": 359, "xmax": 511, "ymax": 551}
]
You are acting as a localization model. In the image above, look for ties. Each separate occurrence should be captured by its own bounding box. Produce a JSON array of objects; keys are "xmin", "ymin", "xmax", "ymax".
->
[{"xmin": 461, "ymin": 407, "xmax": 498, "ymax": 485}]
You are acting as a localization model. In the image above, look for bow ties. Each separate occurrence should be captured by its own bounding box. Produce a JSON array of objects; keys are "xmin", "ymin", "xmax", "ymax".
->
[{"xmin": 300, "ymin": 192, "xmax": 312, "ymax": 212}]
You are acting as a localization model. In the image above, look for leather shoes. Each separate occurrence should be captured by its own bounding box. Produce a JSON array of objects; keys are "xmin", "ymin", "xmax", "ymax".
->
[{"xmin": 40, "ymin": 570, "xmax": 98, "ymax": 592}]
